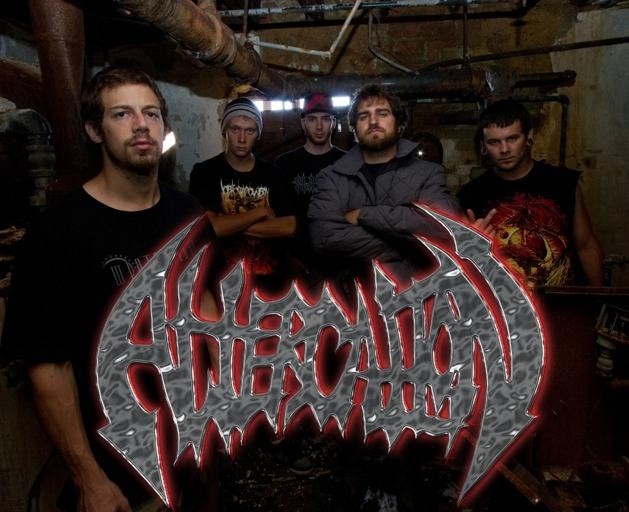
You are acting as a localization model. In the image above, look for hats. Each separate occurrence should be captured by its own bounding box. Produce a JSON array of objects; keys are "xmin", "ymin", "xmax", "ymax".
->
[
  {"xmin": 300, "ymin": 91, "xmax": 337, "ymax": 119},
  {"xmin": 221, "ymin": 97, "xmax": 264, "ymax": 141}
]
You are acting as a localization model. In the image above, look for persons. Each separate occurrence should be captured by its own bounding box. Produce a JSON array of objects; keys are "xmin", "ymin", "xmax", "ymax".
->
[
  {"xmin": 455, "ymin": 100, "xmax": 606, "ymax": 509},
  {"xmin": 272, "ymin": 93, "xmax": 351, "ymax": 480},
  {"xmin": 189, "ymin": 97, "xmax": 298, "ymax": 483},
  {"xmin": 308, "ymin": 84, "xmax": 464, "ymax": 510},
  {"xmin": 0, "ymin": 63, "xmax": 226, "ymax": 512},
  {"xmin": 411, "ymin": 131, "xmax": 445, "ymax": 164}
]
